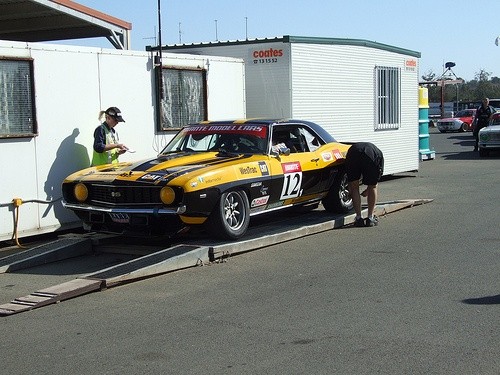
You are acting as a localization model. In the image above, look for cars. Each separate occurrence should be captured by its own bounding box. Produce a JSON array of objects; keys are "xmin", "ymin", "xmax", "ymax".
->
[
  {"xmin": 437, "ymin": 109, "xmax": 478, "ymax": 133},
  {"xmin": 478, "ymin": 111, "xmax": 500, "ymax": 156},
  {"xmin": 488, "ymin": 99, "xmax": 500, "ymax": 114}
]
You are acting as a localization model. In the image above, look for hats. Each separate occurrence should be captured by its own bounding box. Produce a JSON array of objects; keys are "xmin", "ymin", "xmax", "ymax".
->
[{"xmin": 105, "ymin": 107, "xmax": 125, "ymax": 122}]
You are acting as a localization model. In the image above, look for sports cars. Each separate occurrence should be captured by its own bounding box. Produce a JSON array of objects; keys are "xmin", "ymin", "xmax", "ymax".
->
[{"xmin": 61, "ymin": 118, "xmax": 363, "ymax": 242}]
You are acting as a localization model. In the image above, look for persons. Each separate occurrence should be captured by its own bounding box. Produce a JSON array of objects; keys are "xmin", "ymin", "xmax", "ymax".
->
[
  {"xmin": 91, "ymin": 107, "xmax": 129, "ymax": 167},
  {"xmin": 345, "ymin": 142, "xmax": 384, "ymax": 228},
  {"xmin": 471, "ymin": 98, "xmax": 496, "ymax": 153},
  {"xmin": 274, "ymin": 131, "xmax": 297, "ymax": 153}
]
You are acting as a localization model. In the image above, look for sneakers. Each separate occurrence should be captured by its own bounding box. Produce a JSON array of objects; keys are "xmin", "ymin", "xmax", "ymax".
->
[
  {"xmin": 354, "ymin": 218, "xmax": 364, "ymax": 227},
  {"xmin": 366, "ymin": 217, "xmax": 378, "ymax": 227}
]
